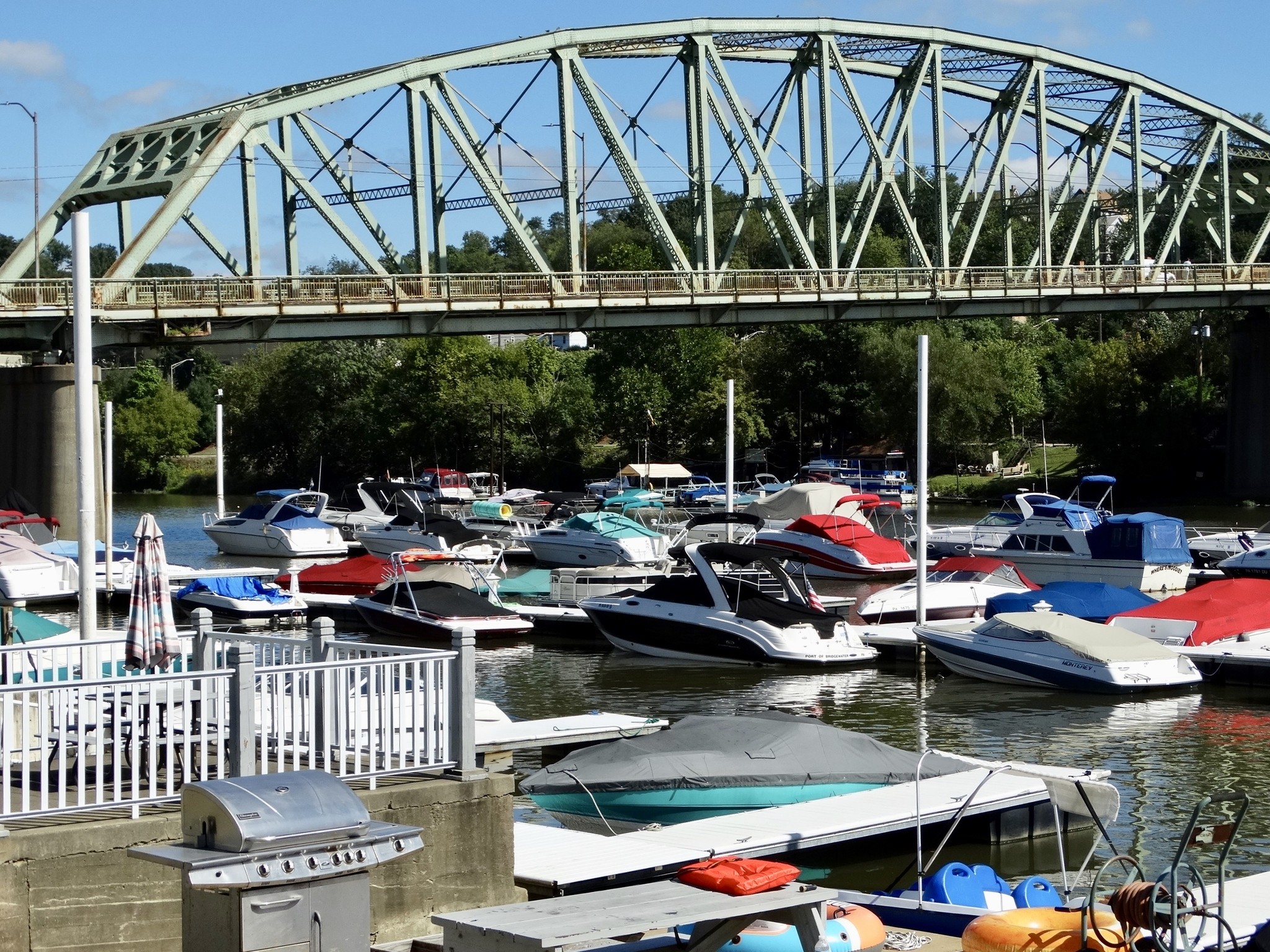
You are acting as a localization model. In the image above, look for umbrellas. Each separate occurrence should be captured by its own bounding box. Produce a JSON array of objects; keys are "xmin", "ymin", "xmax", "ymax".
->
[{"xmin": 125, "ymin": 513, "xmax": 183, "ymax": 672}]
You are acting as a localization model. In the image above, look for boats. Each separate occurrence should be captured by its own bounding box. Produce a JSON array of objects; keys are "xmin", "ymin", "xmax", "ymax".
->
[
  {"xmin": 514, "ymin": 710, "xmax": 977, "ymax": 826},
  {"xmin": 492, "ymin": 565, "xmax": 693, "ymax": 628},
  {"xmin": 735, "ymin": 514, "xmax": 918, "ymax": 582},
  {"xmin": 983, "ymin": 579, "xmax": 1163, "ymax": 621},
  {"xmin": 651, "ymin": 506, "xmax": 796, "ymax": 550},
  {"xmin": 790, "ymin": 450, "xmax": 930, "ymax": 510},
  {"xmin": 350, "ymin": 551, "xmax": 534, "ymax": 640},
  {"xmin": 856, "ymin": 556, "xmax": 1044, "ymax": 625},
  {"xmin": 969, "ymin": 474, "xmax": 1194, "ymax": 592},
  {"xmin": 168, "ymin": 565, "xmax": 308, "ymax": 628},
  {"xmin": 0, "ymin": 508, "xmax": 81, "ymax": 607},
  {"xmin": 851, "ymin": 610, "xmax": 988, "ymax": 663},
  {"xmin": 501, "ymin": 510, "xmax": 672, "ymax": 568},
  {"xmin": 911, "ymin": 598, "xmax": 1202, "ymax": 693},
  {"xmin": 575, "ymin": 542, "xmax": 880, "ymax": 669},
  {"xmin": 1102, "ymin": 575, "xmax": 1270, "ymax": 667},
  {"xmin": 1186, "ymin": 520, "xmax": 1270, "ymax": 564},
  {"xmin": 908, "ymin": 510, "xmax": 1026, "ymax": 560},
  {"xmin": 37, "ymin": 516, "xmax": 196, "ymax": 596},
  {"xmin": 1215, "ymin": 543, "xmax": 1270, "ymax": 578},
  {"xmin": 202, "ymin": 467, "xmax": 788, "ymax": 600},
  {"xmin": 738, "ymin": 481, "xmax": 875, "ymax": 537}
]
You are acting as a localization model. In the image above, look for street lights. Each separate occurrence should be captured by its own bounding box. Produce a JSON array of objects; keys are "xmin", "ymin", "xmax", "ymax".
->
[{"xmin": 542, "ymin": 123, "xmax": 587, "ymax": 272}]
